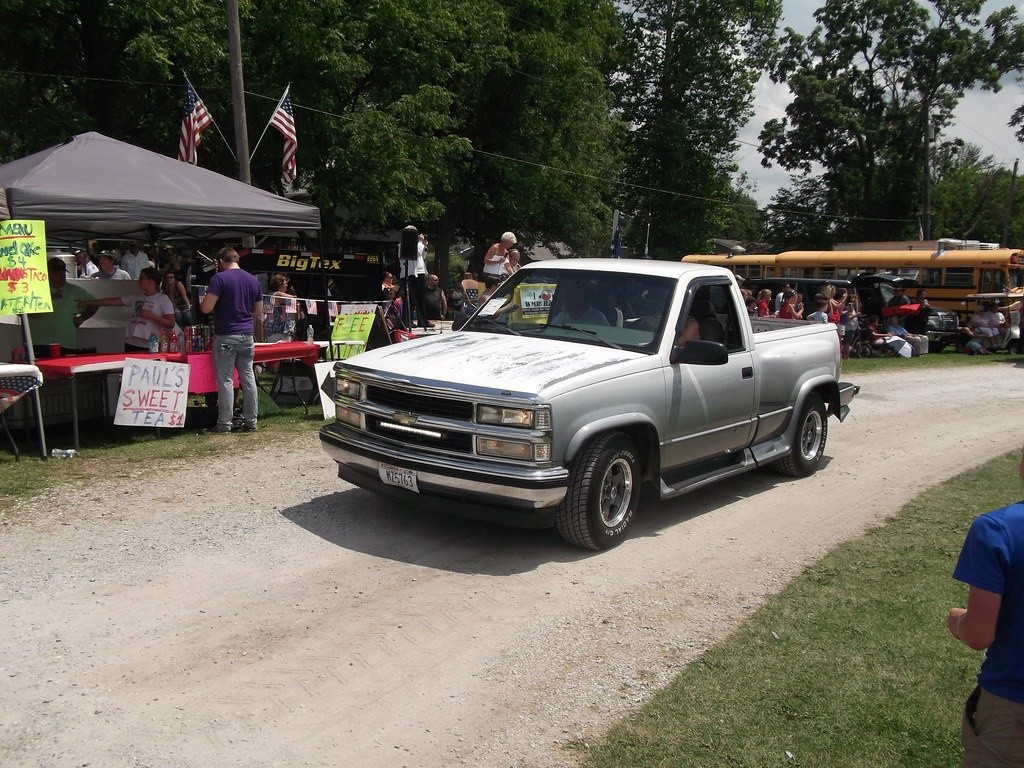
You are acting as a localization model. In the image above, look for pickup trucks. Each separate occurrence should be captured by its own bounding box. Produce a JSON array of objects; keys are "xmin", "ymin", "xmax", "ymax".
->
[{"xmin": 320, "ymin": 258, "xmax": 861, "ymax": 550}]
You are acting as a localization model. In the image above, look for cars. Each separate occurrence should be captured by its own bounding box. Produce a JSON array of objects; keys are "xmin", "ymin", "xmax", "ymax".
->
[{"xmin": 741, "ymin": 276, "xmax": 869, "ymax": 350}]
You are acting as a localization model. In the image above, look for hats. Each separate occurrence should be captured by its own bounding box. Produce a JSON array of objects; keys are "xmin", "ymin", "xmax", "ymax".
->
[
  {"xmin": 48, "ymin": 258, "xmax": 70, "ymax": 273},
  {"xmin": 96, "ymin": 250, "xmax": 119, "ymax": 266}
]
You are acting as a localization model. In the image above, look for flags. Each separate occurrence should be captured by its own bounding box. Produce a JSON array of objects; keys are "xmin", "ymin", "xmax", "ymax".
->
[
  {"xmin": 269, "ymin": 86, "xmax": 297, "ymax": 189},
  {"xmin": 177, "ymin": 83, "xmax": 214, "ymax": 166}
]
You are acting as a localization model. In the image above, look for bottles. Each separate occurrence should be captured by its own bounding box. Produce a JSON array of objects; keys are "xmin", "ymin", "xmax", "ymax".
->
[
  {"xmin": 306, "ymin": 325, "xmax": 314, "ymax": 345},
  {"xmin": 148, "ymin": 330, "xmax": 159, "ymax": 353},
  {"xmin": 160, "ymin": 330, "xmax": 185, "ymax": 353}
]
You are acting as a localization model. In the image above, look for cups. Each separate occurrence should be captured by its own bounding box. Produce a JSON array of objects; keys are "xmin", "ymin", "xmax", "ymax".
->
[{"xmin": 48, "ymin": 343, "xmax": 61, "ymax": 358}]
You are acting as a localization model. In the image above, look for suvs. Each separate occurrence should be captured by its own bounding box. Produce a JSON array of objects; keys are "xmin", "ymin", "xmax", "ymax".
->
[{"xmin": 849, "ymin": 272, "xmax": 962, "ymax": 353}]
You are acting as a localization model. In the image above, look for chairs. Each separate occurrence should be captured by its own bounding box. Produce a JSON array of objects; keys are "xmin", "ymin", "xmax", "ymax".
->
[
  {"xmin": 692, "ymin": 300, "xmax": 725, "ymax": 344},
  {"xmin": 595, "ymin": 289, "xmax": 622, "ymax": 327}
]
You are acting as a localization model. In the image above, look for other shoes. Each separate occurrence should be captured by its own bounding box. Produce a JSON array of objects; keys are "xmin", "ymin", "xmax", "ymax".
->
[
  {"xmin": 203, "ymin": 426, "xmax": 231, "ymax": 434},
  {"xmin": 237, "ymin": 425, "xmax": 258, "ymax": 431}
]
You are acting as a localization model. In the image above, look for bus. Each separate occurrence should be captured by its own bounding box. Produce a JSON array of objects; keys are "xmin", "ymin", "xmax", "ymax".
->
[{"xmin": 680, "ymin": 239, "xmax": 1024, "ymax": 354}]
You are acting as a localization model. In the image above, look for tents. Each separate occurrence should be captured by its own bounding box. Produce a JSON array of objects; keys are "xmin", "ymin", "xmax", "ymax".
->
[{"xmin": 0, "ymin": 132, "xmax": 323, "ymax": 465}]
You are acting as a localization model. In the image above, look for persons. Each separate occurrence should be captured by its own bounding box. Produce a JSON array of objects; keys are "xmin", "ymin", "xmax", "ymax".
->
[
  {"xmin": 549, "ymin": 280, "xmax": 610, "ymax": 327},
  {"xmin": 635, "ymin": 290, "xmax": 702, "ymax": 362},
  {"xmin": 945, "ymin": 440, "xmax": 1023, "ymax": 767},
  {"xmin": 19, "ymin": 256, "xmax": 100, "ymax": 358},
  {"xmin": 76, "ymin": 268, "xmax": 176, "ymax": 352},
  {"xmin": 735, "ymin": 274, "xmax": 1024, "ymax": 364},
  {"xmin": 27, "ymin": 225, "xmax": 524, "ymax": 433},
  {"xmin": 199, "ymin": 245, "xmax": 263, "ymax": 434}
]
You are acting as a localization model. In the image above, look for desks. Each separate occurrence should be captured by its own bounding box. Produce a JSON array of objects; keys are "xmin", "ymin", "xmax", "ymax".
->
[{"xmin": 10, "ymin": 340, "xmax": 320, "ymax": 453}]
